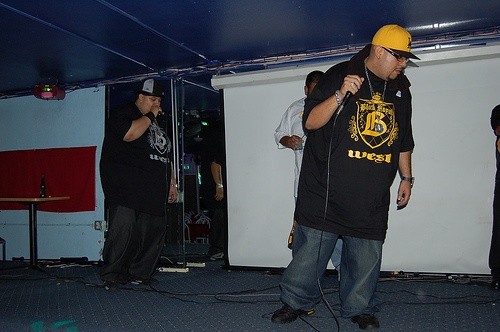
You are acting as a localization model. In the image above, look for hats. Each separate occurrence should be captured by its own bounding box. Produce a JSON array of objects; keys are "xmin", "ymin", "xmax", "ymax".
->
[
  {"xmin": 136, "ymin": 78, "xmax": 165, "ymax": 97},
  {"xmin": 372, "ymin": 24, "xmax": 421, "ymax": 60}
]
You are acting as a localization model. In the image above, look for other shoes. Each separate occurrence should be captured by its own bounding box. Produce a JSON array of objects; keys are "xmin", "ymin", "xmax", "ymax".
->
[
  {"xmin": 103, "ymin": 279, "xmax": 117, "ymax": 291},
  {"xmin": 350, "ymin": 313, "xmax": 381, "ymax": 332},
  {"xmin": 271, "ymin": 302, "xmax": 315, "ymax": 323}
]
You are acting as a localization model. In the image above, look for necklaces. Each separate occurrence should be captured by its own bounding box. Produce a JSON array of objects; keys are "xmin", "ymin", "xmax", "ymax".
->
[{"xmin": 364, "ymin": 57, "xmax": 387, "ymax": 133}]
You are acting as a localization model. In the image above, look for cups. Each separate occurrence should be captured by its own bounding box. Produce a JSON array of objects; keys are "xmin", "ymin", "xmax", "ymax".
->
[{"xmin": 294, "ymin": 137, "xmax": 302, "ymax": 150}]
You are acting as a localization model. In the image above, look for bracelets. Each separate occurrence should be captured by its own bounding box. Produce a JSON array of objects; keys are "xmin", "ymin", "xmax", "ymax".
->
[
  {"xmin": 216, "ymin": 183, "xmax": 223, "ymax": 189},
  {"xmin": 144, "ymin": 112, "xmax": 156, "ymax": 123},
  {"xmin": 170, "ymin": 183, "xmax": 178, "ymax": 188},
  {"xmin": 335, "ymin": 90, "xmax": 341, "ymax": 106}
]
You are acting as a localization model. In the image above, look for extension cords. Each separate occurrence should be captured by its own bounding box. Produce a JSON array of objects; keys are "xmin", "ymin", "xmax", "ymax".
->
[
  {"xmin": 177, "ymin": 262, "xmax": 205, "ymax": 267},
  {"xmin": 158, "ymin": 267, "xmax": 189, "ymax": 272}
]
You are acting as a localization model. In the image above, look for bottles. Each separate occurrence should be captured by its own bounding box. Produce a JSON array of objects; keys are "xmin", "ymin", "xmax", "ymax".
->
[{"xmin": 39, "ymin": 176, "xmax": 46, "ymax": 198}]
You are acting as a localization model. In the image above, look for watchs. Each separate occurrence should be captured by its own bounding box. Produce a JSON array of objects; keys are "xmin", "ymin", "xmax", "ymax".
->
[{"xmin": 401, "ymin": 176, "xmax": 414, "ymax": 183}]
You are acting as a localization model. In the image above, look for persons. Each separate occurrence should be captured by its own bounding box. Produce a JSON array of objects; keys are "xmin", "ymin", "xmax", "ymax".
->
[
  {"xmin": 202, "ymin": 117, "xmax": 229, "ymax": 259},
  {"xmin": 270, "ymin": 24, "xmax": 420, "ymax": 332},
  {"xmin": 489, "ymin": 103, "xmax": 500, "ymax": 286},
  {"xmin": 99, "ymin": 78, "xmax": 178, "ymax": 291},
  {"xmin": 274, "ymin": 70, "xmax": 344, "ymax": 283}
]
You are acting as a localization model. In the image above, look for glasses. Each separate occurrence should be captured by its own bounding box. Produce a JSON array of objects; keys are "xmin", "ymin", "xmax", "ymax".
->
[{"xmin": 381, "ymin": 47, "xmax": 403, "ymax": 61}]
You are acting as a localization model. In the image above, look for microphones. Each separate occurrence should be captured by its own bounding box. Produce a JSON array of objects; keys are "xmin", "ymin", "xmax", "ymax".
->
[{"xmin": 337, "ymin": 91, "xmax": 352, "ymax": 116}]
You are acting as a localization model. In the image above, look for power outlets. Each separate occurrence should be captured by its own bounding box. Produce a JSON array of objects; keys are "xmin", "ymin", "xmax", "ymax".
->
[{"xmin": 94, "ymin": 220, "xmax": 102, "ymax": 230}]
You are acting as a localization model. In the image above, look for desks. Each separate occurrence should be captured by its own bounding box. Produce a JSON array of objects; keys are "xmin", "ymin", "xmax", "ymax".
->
[{"xmin": 0, "ymin": 196, "xmax": 71, "ymax": 274}]
